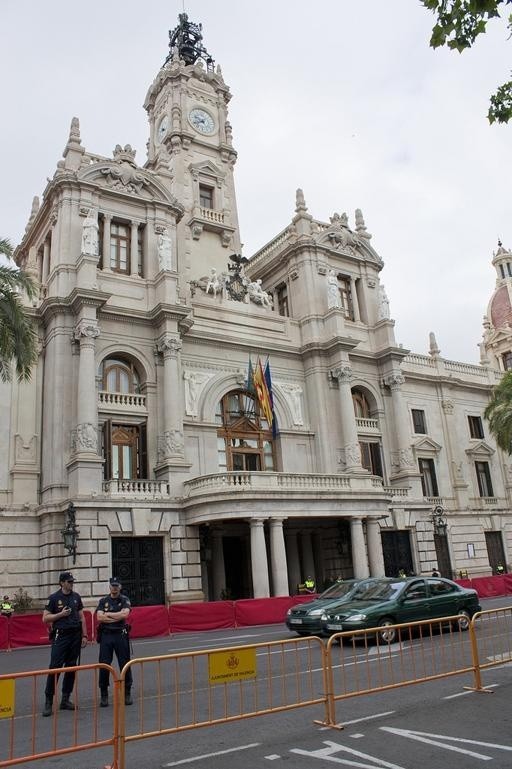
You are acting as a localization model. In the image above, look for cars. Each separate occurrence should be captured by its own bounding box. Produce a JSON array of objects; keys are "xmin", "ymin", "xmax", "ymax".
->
[{"xmin": 286, "ymin": 576, "xmax": 482, "ymax": 645}]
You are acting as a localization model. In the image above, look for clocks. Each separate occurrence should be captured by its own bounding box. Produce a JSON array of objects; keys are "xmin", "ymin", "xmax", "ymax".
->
[
  {"xmin": 187, "ymin": 105, "xmax": 219, "ymax": 136},
  {"xmin": 155, "ymin": 110, "xmax": 173, "ymax": 144}
]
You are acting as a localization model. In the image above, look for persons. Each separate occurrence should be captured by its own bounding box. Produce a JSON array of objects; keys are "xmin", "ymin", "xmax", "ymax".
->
[
  {"xmin": 206, "ymin": 268, "xmax": 219, "ymax": 299},
  {"xmin": 303, "ymin": 576, "xmax": 315, "ymax": 595},
  {"xmin": 0, "ymin": 595, "xmax": 14, "ymax": 619},
  {"xmin": 496, "ymin": 561, "xmax": 505, "ymax": 575},
  {"xmin": 96, "ymin": 577, "xmax": 133, "ymax": 706},
  {"xmin": 335, "ymin": 576, "xmax": 344, "ymax": 584},
  {"xmin": 398, "ymin": 568, "xmax": 438, "ymax": 577},
  {"xmin": 42, "ymin": 572, "xmax": 87, "ymax": 716},
  {"xmin": 248, "ymin": 279, "xmax": 272, "ymax": 308}
]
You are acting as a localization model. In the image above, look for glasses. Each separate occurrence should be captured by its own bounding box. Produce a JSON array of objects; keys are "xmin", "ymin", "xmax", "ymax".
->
[{"xmin": 67, "ymin": 580, "xmax": 74, "ymax": 583}]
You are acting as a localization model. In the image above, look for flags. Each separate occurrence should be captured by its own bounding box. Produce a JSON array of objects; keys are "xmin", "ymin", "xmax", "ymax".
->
[{"xmin": 247, "ymin": 359, "xmax": 276, "ymax": 440}]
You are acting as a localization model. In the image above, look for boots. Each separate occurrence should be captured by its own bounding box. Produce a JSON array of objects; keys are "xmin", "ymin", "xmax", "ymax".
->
[
  {"xmin": 125, "ymin": 686, "xmax": 133, "ymax": 706},
  {"xmin": 100, "ymin": 687, "xmax": 108, "ymax": 707},
  {"xmin": 43, "ymin": 696, "xmax": 53, "ymax": 716},
  {"xmin": 60, "ymin": 692, "xmax": 78, "ymax": 710}
]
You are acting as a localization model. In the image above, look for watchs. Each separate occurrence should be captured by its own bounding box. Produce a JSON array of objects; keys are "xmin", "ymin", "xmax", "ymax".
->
[{"xmin": 82, "ymin": 635, "xmax": 88, "ymax": 639}]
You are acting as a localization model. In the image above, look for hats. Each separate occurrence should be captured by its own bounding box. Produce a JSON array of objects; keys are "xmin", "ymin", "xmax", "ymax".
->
[
  {"xmin": 109, "ymin": 577, "xmax": 120, "ymax": 586},
  {"xmin": 3, "ymin": 595, "xmax": 10, "ymax": 600},
  {"xmin": 59, "ymin": 572, "xmax": 77, "ymax": 581}
]
